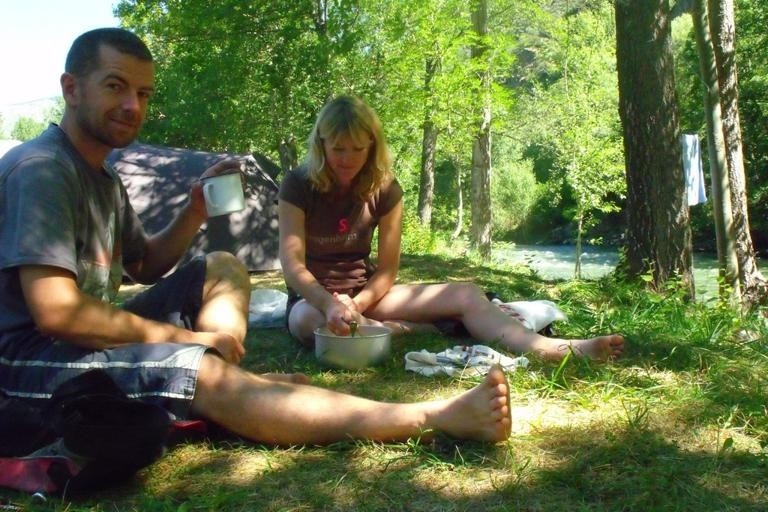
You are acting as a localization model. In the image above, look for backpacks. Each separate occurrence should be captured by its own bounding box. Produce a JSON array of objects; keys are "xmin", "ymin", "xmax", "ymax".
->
[{"xmin": 1, "ymin": 370, "xmax": 170, "ymax": 497}]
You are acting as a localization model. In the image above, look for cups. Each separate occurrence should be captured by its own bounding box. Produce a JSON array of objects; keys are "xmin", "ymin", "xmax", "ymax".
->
[{"xmin": 200, "ymin": 172, "xmax": 247, "ymax": 219}]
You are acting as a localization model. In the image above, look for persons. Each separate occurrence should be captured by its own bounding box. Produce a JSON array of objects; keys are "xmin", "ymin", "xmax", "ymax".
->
[
  {"xmin": 0, "ymin": 27, "xmax": 512, "ymax": 447},
  {"xmin": 278, "ymin": 96, "xmax": 625, "ymax": 360}
]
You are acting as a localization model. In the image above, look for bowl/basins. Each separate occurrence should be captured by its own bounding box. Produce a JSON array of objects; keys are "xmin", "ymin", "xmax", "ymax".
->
[{"xmin": 314, "ymin": 324, "xmax": 393, "ymax": 369}]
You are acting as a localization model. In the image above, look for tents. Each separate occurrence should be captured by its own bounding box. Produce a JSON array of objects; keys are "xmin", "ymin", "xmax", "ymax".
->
[{"xmin": 105, "ymin": 143, "xmax": 278, "ymax": 282}]
